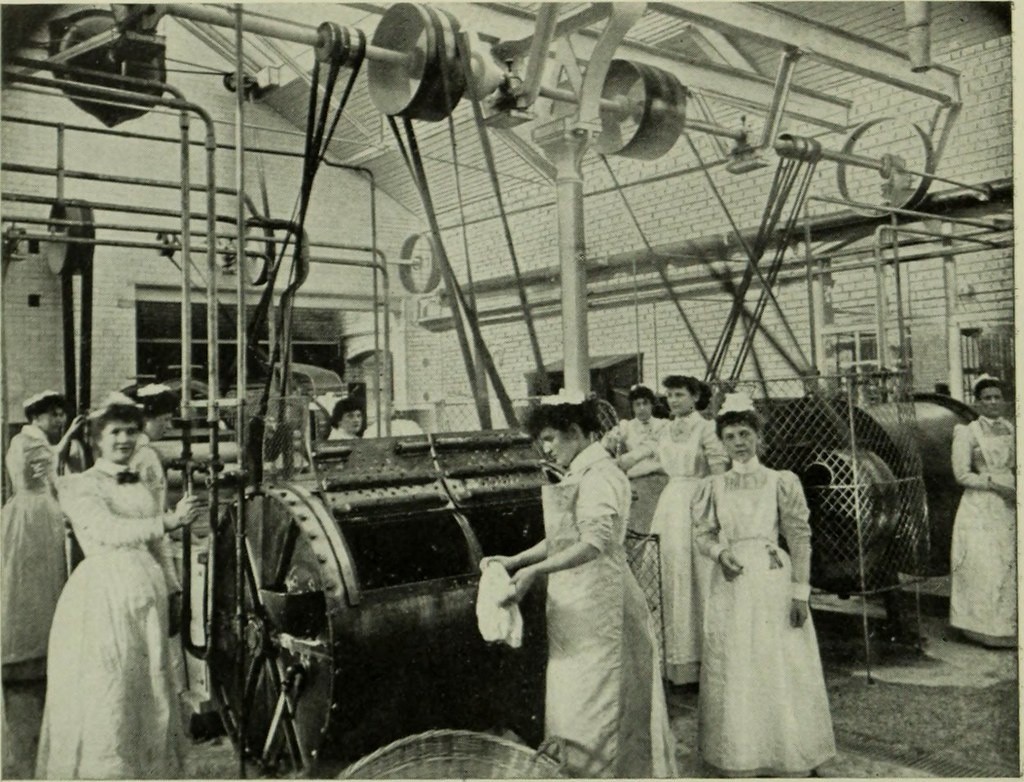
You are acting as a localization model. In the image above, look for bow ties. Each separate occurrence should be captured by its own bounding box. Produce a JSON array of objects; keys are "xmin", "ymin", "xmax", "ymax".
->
[{"xmin": 117, "ymin": 470, "xmax": 139, "ymax": 485}]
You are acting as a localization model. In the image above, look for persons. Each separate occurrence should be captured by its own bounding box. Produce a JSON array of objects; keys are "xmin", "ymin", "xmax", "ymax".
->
[
  {"xmin": 690, "ymin": 392, "xmax": 837, "ymax": 779},
  {"xmin": 0, "ymin": 388, "xmax": 86, "ymax": 668},
  {"xmin": 615, "ymin": 374, "xmax": 728, "ymax": 684},
  {"xmin": 597, "ymin": 384, "xmax": 673, "ymax": 568},
  {"xmin": 122, "ymin": 382, "xmax": 183, "ymax": 701},
  {"xmin": 949, "ymin": 373, "xmax": 1016, "ymax": 650},
  {"xmin": 32, "ymin": 390, "xmax": 202, "ymax": 780},
  {"xmin": 328, "ymin": 397, "xmax": 363, "ymax": 440},
  {"xmin": 476, "ymin": 387, "xmax": 679, "ymax": 779}
]
